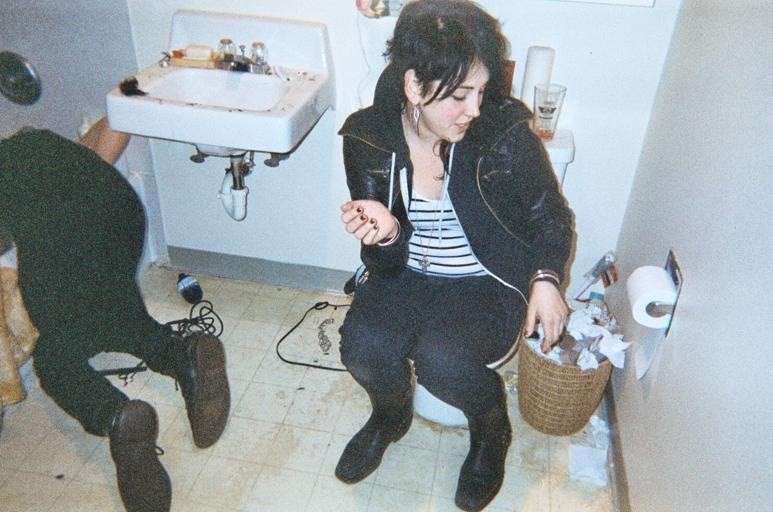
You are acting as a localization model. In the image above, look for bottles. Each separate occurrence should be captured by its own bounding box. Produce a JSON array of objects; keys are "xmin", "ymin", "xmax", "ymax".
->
[{"xmin": 176, "ymin": 272, "xmax": 204, "ymax": 304}]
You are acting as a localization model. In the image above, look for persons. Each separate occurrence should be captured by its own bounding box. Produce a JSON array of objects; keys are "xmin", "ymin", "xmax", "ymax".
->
[
  {"xmin": 335, "ymin": 2, "xmax": 572, "ymax": 512},
  {"xmin": 0, "ymin": 125, "xmax": 231, "ymax": 511}
]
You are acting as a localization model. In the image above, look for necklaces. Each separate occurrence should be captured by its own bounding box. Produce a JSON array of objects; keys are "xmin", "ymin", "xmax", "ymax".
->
[{"xmin": 410, "ymin": 168, "xmax": 447, "ymax": 282}]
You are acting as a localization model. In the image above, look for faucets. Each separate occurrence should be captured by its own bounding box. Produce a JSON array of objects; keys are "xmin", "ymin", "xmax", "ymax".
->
[{"xmin": 220, "ymin": 57, "xmax": 247, "ymax": 73}]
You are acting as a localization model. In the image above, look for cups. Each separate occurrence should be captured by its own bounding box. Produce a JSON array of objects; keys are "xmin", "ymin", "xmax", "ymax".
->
[{"xmin": 532, "ymin": 82, "xmax": 567, "ymax": 141}]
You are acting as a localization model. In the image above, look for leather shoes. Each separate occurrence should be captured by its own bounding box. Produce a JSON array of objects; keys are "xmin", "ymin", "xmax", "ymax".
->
[
  {"xmin": 456, "ymin": 415, "xmax": 512, "ymax": 511},
  {"xmin": 335, "ymin": 407, "xmax": 412, "ymax": 484}
]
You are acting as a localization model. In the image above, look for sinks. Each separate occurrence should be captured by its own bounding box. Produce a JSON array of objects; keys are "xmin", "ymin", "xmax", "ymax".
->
[{"xmin": 105, "ymin": 58, "xmax": 330, "ymax": 155}]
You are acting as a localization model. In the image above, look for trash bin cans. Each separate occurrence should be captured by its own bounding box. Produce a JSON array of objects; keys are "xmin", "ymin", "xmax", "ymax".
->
[{"xmin": 519, "ymin": 303, "xmax": 615, "ymax": 435}]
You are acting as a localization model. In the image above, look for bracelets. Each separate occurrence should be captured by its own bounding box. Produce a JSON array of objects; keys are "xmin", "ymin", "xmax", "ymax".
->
[{"xmin": 526, "ymin": 267, "xmax": 560, "ymax": 286}]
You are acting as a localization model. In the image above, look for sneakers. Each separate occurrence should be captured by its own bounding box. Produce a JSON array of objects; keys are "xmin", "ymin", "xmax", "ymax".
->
[
  {"xmin": 111, "ymin": 399, "xmax": 171, "ymax": 511},
  {"xmin": 166, "ymin": 331, "xmax": 229, "ymax": 449}
]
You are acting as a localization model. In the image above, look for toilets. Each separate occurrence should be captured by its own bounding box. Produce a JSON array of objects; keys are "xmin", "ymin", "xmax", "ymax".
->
[{"xmin": 410, "ymin": 128, "xmax": 574, "ymax": 427}]
